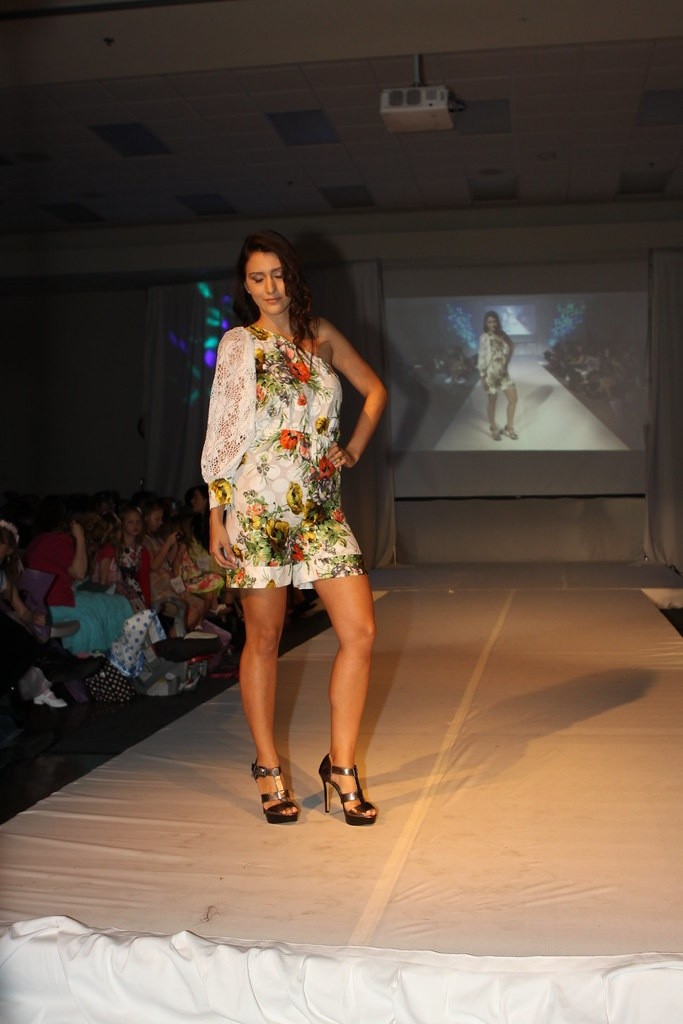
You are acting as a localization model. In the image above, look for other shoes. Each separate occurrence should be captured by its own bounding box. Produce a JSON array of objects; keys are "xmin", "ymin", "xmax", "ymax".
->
[{"xmin": 33, "ymin": 690, "xmax": 66, "ymax": 707}]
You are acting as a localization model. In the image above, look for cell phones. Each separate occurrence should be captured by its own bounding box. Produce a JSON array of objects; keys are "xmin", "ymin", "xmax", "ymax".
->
[{"xmin": 176, "ymin": 528, "xmax": 186, "ymax": 541}]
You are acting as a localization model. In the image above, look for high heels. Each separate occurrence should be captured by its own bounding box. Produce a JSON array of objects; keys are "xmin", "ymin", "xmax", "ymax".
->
[
  {"xmin": 250, "ymin": 753, "xmax": 378, "ymax": 826},
  {"xmin": 489, "ymin": 426, "xmax": 518, "ymax": 441}
]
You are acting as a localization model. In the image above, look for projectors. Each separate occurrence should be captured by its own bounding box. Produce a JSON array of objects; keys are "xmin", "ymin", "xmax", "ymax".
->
[{"xmin": 380, "ymin": 84, "xmax": 454, "ymax": 132}]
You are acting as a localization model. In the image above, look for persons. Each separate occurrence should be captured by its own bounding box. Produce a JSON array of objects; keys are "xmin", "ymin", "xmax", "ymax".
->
[
  {"xmin": 200, "ymin": 232, "xmax": 390, "ymax": 822},
  {"xmin": 399, "ymin": 335, "xmax": 471, "ymax": 415},
  {"xmin": 542, "ymin": 337, "xmax": 645, "ymax": 430},
  {"xmin": 477, "ymin": 310, "xmax": 521, "ymax": 444},
  {"xmin": 2, "ymin": 479, "xmax": 265, "ymax": 711}
]
